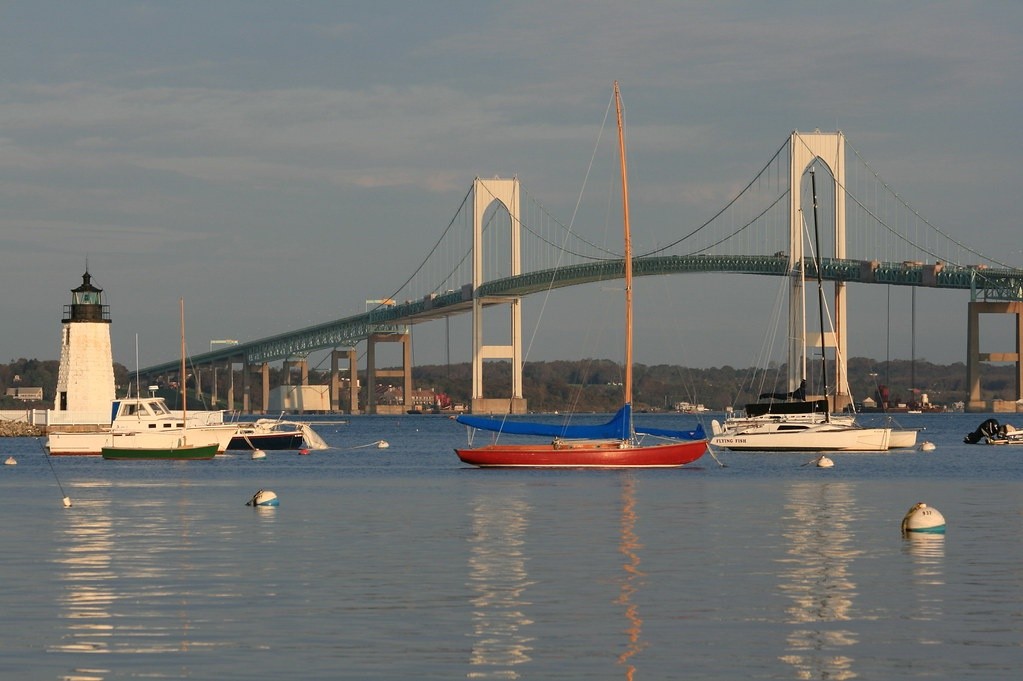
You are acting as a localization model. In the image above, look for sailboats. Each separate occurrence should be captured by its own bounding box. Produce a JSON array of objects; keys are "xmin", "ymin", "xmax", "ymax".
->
[
  {"xmin": 449, "ymin": 79, "xmax": 713, "ymax": 470},
  {"xmin": 707, "ymin": 208, "xmax": 926, "ymax": 452}
]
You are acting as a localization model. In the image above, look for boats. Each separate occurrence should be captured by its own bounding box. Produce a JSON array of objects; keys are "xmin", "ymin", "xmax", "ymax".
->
[
  {"xmin": 41, "ymin": 295, "xmax": 329, "ymax": 459},
  {"xmin": 406, "ymin": 409, "xmax": 433, "ymax": 414},
  {"xmin": 885, "ymin": 405, "xmax": 948, "ymax": 413},
  {"xmin": 962, "ymin": 418, "xmax": 1023, "ymax": 444}
]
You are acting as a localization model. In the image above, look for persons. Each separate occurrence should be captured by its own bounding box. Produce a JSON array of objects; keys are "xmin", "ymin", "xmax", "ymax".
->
[{"xmin": 83, "ymin": 294, "xmax": 91, "ymax": 304}]
